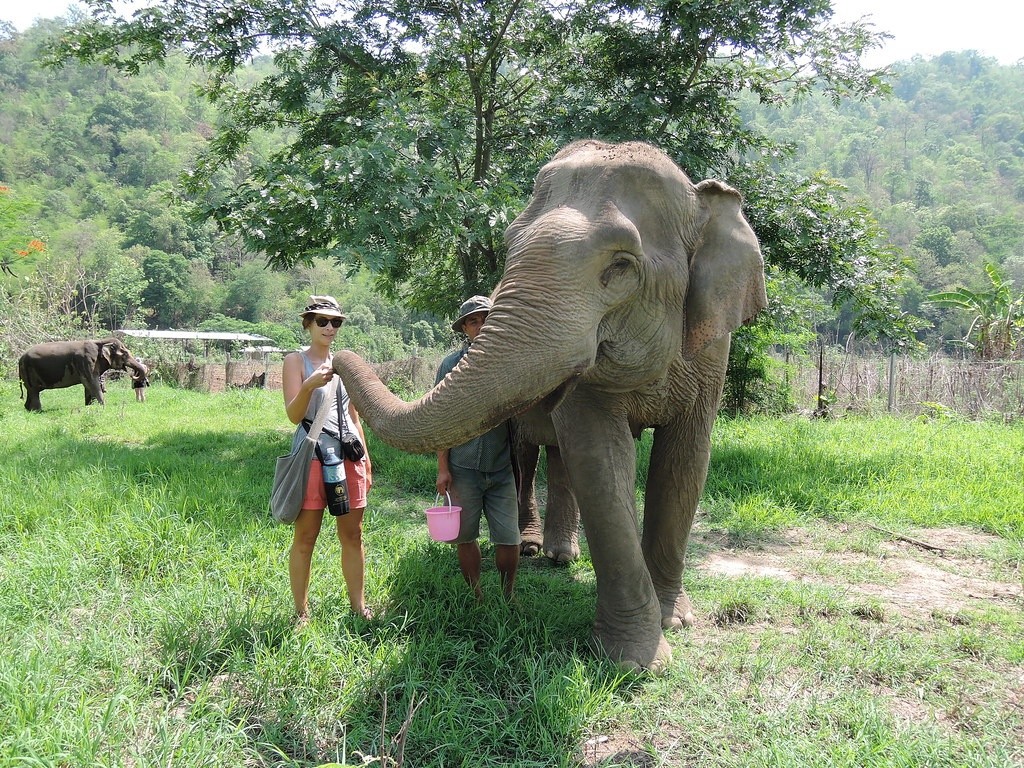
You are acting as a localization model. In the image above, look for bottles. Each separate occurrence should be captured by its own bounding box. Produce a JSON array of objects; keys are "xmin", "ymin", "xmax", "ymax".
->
[{"xmin": 322, "ymin": 447, "xmax": 350, "ymax": 515}]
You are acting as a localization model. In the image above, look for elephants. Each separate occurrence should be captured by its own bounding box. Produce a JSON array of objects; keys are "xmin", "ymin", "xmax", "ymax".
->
[
  {"xmin": 19, "ymin": 337, "xmax": 147, "ymax": 414},
  {"xmin": 329, "ymin": 137, "xmax": 769, "ymax": 680}
]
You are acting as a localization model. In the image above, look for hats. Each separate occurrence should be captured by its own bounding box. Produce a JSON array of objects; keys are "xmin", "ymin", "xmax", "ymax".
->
[
  {"xmin": 299, "ymin": 296, "xmax": 346, "ymax": 320},
  {"xmin": 452, "ymin": 295, "xmax": 494, "ymax": 332}
]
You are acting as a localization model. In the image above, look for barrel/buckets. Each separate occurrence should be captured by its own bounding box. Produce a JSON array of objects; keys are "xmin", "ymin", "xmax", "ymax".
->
[{"xmin": 425, "ymin": 491, "xmax": 462, "ymax": 540}]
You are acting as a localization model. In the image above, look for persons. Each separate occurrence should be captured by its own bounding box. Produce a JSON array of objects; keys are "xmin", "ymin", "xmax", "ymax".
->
[
  {"xmin": 130, "ymin": 357, "xmax": 150, "ymax": 403},
  {"xmin": 433, "ymin": 295, "xmax": 525, "ymax": 619},
  {"xmin": 282, "ymin": 295, "xmax": 374, "ymax": 631}
]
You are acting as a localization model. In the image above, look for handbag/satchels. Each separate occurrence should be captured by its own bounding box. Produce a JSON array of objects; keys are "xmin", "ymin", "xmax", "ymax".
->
[{"xmin": 271, "ymin": 437, "xmax": 316, "ymax": 525}]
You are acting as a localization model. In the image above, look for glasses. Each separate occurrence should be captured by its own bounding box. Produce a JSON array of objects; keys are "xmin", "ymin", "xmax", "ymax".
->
[{"xmin": 313, "ymin": 317, "xmax": 343, "ymax": 328}]
